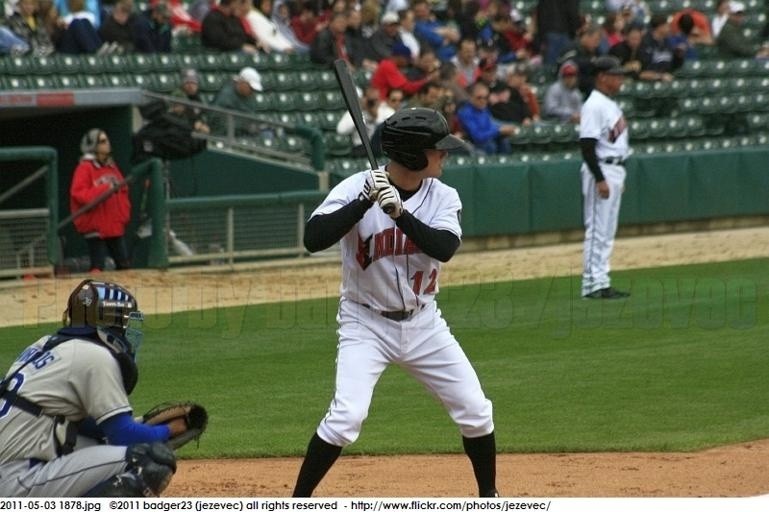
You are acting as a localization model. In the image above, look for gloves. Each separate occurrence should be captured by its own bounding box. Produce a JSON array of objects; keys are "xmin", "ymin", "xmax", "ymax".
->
[
  {"xmin": 361, "ymin": 169, "xmax": 390, "ymax": 203},
  {"xmin": 376, "ymin": 185, "xmax": 404, "ymax": 220}
]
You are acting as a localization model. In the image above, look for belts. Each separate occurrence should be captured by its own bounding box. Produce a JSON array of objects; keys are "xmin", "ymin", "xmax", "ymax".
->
[
  {"xmin": 363, "ymin": 304, "xmax": 425, "ymax": 321},
  {"xmin": 606, "ymin": 160, "xmax": 627, "ymax": 166}
]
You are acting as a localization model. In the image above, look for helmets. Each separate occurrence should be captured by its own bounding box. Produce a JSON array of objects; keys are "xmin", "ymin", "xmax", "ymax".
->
[
  {"xmin": 381, "ymin": 107, "xmax": 466, "ymax": 171},
  {"xmin": 67, "ymin": 278, "xmax": 146, "ymax": 362}
]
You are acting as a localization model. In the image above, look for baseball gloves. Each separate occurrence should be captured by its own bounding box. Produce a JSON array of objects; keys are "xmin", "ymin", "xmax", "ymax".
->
[{"xmin": 142, "ymin": 400, "xmax": 207, "ymax": 450}]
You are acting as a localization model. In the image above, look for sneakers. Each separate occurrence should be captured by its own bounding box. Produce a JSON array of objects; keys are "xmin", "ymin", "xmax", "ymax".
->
[{"xmin": 582, "ymin": 287, "xmax": 629, "ymax": 300}]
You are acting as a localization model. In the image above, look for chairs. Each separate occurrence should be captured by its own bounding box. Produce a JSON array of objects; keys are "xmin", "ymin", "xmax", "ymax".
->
[
  {"xmin": 1, "ymin": 47, "xmax": 372, "ymax": 91},
  {"xmin": 508, "ymin": 0, "xmax": 768, "ymax": 151},
  {"xmin": 180, "ymin": 91, "xmax": 376, "ymax": 175}
]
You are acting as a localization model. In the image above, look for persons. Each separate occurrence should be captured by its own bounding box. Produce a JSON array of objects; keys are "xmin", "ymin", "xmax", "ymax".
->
[
  {"xmin": 0, "ymin": 278, "xmax": 209, "ymax": 498},
  {"xmin": 292, "ymin": 106, "xmax": 501, "ymax": 499},
  {"xmin": 577, "ymin": 55, "xmax": 635, "ymax": 302},
  {"xmin": 68, "ymin": 127, "xmax": 133, "ymax": 275},
  {"xmin": 0, "ymin": 2, "xmax": 767, "ymax": 160}
]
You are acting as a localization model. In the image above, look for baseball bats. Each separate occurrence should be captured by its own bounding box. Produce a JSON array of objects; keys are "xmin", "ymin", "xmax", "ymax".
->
[{"xmin": 334, "ymin": 60, "xmax": 396, "ymax": 214}]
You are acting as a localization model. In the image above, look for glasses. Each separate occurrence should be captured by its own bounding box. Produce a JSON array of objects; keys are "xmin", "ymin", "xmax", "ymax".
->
[
  {"xmin": 97, "ymin": 139, "xmax": 108, "ymax": 144},
  {"xmin": 390, "ymin": 97, "xmax": 402, "ymax": 102},
  {"xmin": 476, "ymin": 96, "xmax": 487, "ymax": 100}
]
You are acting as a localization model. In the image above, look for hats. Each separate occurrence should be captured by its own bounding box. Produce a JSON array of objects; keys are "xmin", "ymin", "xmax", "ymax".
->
[
  {"xmin": 730, "ymin": 3, "xmax": 747, "ymax": 15},
  {"xmin": 586, "ymin": 55, "xmax": 628, "ymax": 79},
  {"xmin": 479, "ymin": 59, "xmax": 496, "ymax": 73},
  {"xmin": 559, "ymin": 62, "xmax": 575, "ymax": 76},
  {"xmin": 79, "ymin": 128, "xmax": 99, "ymax": 152},
  {"xmin": 240, "ymin": 66, "xmax": 263, "ymax": 92},
  {"xmin": 180, "ymin": 68, "xmax": 201, "ymax": 86},
  {"xmin": 380, "ymin": 12, "xmax": 399, "ymax": 25}
]
